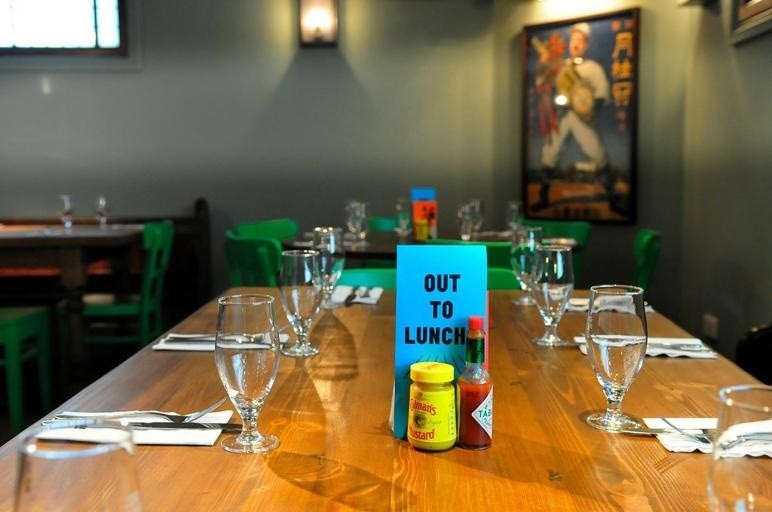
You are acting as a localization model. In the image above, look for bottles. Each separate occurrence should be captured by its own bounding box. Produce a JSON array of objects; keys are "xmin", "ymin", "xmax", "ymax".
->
[{"xmin": 408, "ymin": 316, "xmax": 496, "ymax": 453}]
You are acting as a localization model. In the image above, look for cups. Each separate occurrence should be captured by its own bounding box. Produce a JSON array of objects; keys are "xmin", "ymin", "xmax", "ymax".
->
[
  {"xmin": 10, "ymin": 421, "xmax": 140, "ymax": 512},
  {"xmin": 345, "ymin": 199, "xmax": 521, "ymax": 240},
  {"xmin": 709, "ymin": 386, "xmax": 771, "ymax": 512}
]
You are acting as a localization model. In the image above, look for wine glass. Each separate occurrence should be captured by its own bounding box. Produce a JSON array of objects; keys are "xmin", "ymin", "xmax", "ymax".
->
[
  {"xmin": 56, "ymin": 193, "xmax": 75, "ymax": 230},
  {"xmin": 507, "ymin": 226, "xmax": 650, "ymax": 432},
  {"xmin": 217, "ymin": 226, "xmax": 342, "ymax": 453},
  {"xmin": 94, "ymin": 191, "xmax": 111, "ymax": 230}
]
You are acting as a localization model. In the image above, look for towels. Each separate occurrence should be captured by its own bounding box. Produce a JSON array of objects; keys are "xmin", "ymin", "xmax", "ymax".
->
[
  {"xmin": 573, "ymin": 334, "xmax": 717, "ymax": 359},
  {"xmin": 641, "ymin": 416, "xmax": 772, "ymax": 460}
]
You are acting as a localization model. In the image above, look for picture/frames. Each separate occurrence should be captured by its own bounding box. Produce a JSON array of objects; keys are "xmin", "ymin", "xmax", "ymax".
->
[{"xmin": 521, "ymin": 8, "xmax": 639, "ymax": 225}]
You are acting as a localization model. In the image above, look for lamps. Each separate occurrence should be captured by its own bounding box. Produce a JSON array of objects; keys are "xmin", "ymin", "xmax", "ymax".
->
[{"xmin": 298, "ymin": 0, "xmax": 338, "ymax": 47}]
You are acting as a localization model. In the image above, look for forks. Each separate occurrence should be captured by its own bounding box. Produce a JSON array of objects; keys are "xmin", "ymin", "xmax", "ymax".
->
[
  {"xmin": 54, "ymin": 396, "xmax": 228, "ymax": 423},
  {"xmin": 360, "ymin": 286, "xmax": 374, "ymax": 299}
]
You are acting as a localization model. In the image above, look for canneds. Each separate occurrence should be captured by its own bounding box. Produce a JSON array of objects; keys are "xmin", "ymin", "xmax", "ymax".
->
[{"xmin": 407, "ymin": 362, "xmax": 457, "ymax": 450}]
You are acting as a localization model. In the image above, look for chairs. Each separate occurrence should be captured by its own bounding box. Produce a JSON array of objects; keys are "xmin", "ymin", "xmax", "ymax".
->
[
  {"xmin": 630, "ymin": 227, "xmax": 661, "ymax": 313},
  {"xmin": 144, "ymin": 222, "xmax": 172, "ymax": 254},
  {"xmin": 486, "ymin": 268, "xmax": 528, "ymax": 288},
  {"xmin": 235, "ymin": 217, "xmax": 297, "ymax": 256},
  {"xmin": 222, "ymin": 230, "xmax": 283, "ymax": 287},
  {"xmin": 84, "ymin": 231, "xmax": 173, "ymax": 354},
  {"xmin": 169, "ymin": 196, "xmax": 213, "ymax": 285},
  {"xmin": 1, "ymin": 299, "xmax": 70, "ymax": 436},
  {"xmin": 518, "ymin": 218, "xmax": 593, "ymax": 285},
  {"xmin": 428, "ymin": 238, "xmax": 520, "ymax": 267},
  {"xmin": 361, "ymin": 216, "xmax": 413, "ymax": 236},
  {"xmin": 319, "ymin": 267, "xmax": 396, "ymax": 288}
]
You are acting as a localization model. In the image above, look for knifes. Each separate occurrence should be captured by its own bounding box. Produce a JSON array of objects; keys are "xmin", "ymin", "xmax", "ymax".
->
[
  {"xmin": 41, "ymin": 420, "xmax": 256, "ymax": 439},
  {"xmin": 345, "ymin": 283, "xmax": 360, "ymax": 305}
]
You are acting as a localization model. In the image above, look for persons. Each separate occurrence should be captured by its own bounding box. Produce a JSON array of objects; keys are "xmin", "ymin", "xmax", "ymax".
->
[{"xmin": 527, "ymin": 19, "xmax": 628, "ymax": 219}]
